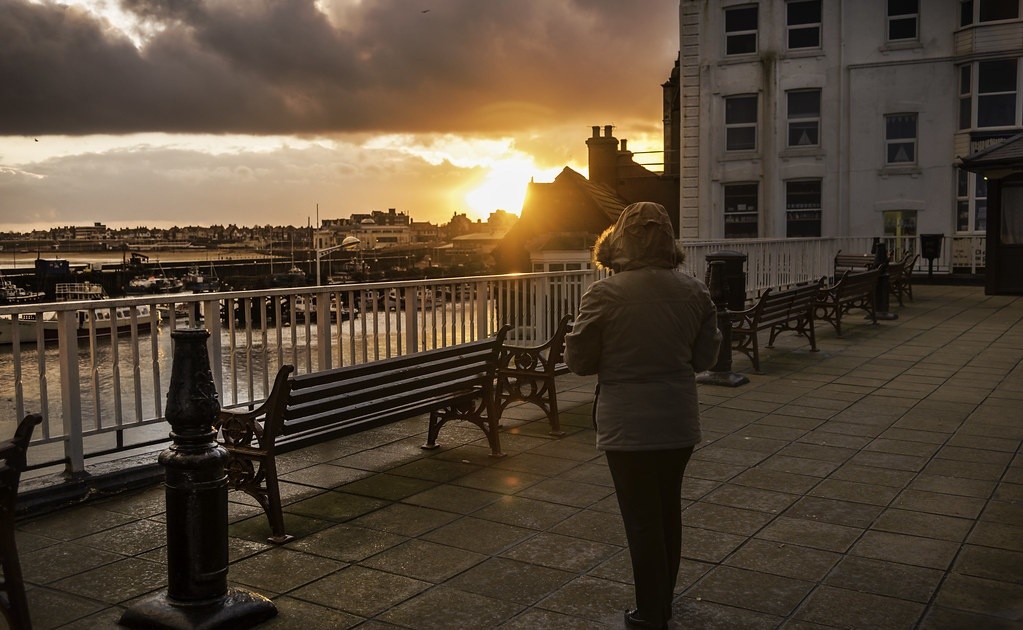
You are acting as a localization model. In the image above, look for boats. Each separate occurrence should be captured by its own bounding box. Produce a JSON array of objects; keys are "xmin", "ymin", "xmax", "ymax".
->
[
  {"xmin": 294, "ymin": 293, "xmax": 358, "ymax": 322},
  {"xmin": 378, "ymin": 289, "xmax": 404, "ymax": 309},
  {"xmin": 0, "ymin": 283, "xmax": 163, "ymax": 343},
  {"xmin": 121, "ymin": 263, "xmax": 183, "ymax": 296},
  {"xmin": 456, "ymin": 286, "xmax": 470, "ymax": 298},
  {"xmin": 0, "ymin": 280, "xmax": 45, "ymax": 304},
  {"xmin": 417, "ymin": 289, "xmax": 442, "ymax": 309}
]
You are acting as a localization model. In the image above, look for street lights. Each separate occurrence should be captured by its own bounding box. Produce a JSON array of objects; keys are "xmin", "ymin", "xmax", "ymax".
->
[
  {"xmin": 412, "ymin": 254, "xmax": 415, "ymax": 269},
  {"xmin": 362, "ymin": 260, "xmax": 365, "ymax": 275},
  {"xmin": 429, "ymin": 258, "xmax": 431, "ymax": 271},
  {"xmin": 406, "ymin": 256, "xmax": 408, "ymax": 273},
  {"xmin": 317, "ymin": 204, "xmax": 361, "ymax": 286}
]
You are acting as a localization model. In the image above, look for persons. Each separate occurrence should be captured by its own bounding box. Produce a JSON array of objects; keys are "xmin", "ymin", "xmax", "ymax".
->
[
  {"xmin": 563, "ymin": 201, "xmax": 721, "ymax": 630},
  {"xmin": 483, "ymin": 221, "xmax": 534, "ymax": 328}
]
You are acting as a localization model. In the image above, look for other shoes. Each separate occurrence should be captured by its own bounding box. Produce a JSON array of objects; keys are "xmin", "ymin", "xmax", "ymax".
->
[{"xmin": 625, "ymin": 607, "xmax": 669, "ymax": 630}]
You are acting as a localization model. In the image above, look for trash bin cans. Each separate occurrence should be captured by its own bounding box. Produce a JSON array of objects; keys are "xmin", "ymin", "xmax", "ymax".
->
[
  {"xmin": 920, "ymin": 233, "xmax": 944, "ymax": 258},
  {"xmin": 705, "ymin": 249, "xmax": 747, "ymax": 321}
]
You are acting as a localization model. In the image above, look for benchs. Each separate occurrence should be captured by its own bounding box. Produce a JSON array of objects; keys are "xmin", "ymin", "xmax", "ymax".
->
[
  {"xmin": 0, "ymin": 413, "xmax": 44, "ymax": 630},
  {"xmin": 794, "ymin": 263, "xmax": 888, "ymax": 339},
  {"xmin": 214, "ymin": 324, "xmax": 514, "ymax": 545},
  {"xmin": 487, "ymin": 313, "xmax": 573, "ymax": 435},
  {"xmin": 834, "ymin": 248, "xmax": 920, "ymax": 309},
  {"xmin": 728, "ymin": 275, "xmax": 828, "ymax": 374}
]
